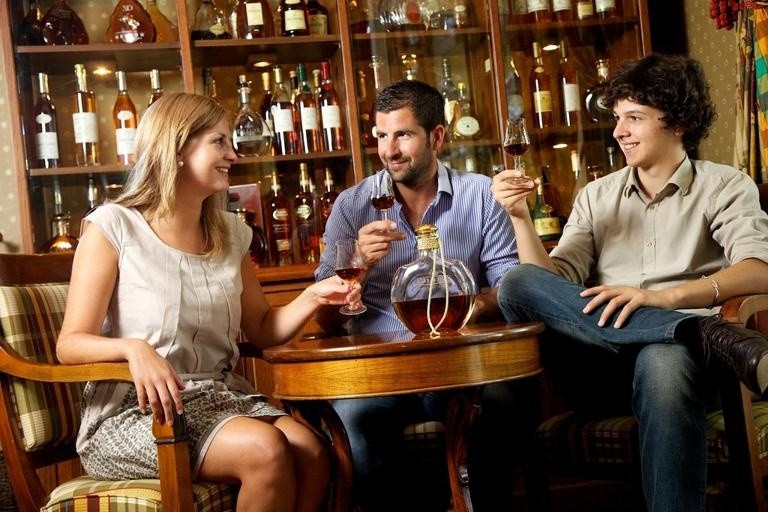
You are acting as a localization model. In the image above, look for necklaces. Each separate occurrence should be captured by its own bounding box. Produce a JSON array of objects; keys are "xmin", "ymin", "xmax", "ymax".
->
[{"xmin": 177, "ymin": 218, "xmax": 210, "ymax": 255}]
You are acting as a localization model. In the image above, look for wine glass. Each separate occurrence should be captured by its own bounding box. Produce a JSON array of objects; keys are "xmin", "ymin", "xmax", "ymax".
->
[{"xmin": 329, "ymin": 235, "xmax": 372, "ymax": 319}]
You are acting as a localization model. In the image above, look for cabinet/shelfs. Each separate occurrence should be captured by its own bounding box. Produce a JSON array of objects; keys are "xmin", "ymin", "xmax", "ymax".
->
[{"xmin": 0, "ymin": 1, "xmax": 658, "ymax": 428}]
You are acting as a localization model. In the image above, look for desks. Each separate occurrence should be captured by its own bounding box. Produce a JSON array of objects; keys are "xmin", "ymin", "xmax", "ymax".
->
[{"xmin": 259, "ymin": 317, "xmax": 572, "ymax": 512}]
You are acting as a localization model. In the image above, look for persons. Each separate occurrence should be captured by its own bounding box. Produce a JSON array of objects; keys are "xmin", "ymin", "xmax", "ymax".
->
[
  {"xmin": 309, "ymin": 80, "xmax": 530, "ymax": 511},
  {"xmin": 55, "ymin": 92, "xmax": 365, "ymax": 509},
  {"xmin": 489, "ymin": 54, "xmax": 768, "ymax": 511}
]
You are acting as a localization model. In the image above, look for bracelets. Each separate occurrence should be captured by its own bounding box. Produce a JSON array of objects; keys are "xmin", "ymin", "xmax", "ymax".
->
[{"xmin": 703, "ymin": 272, "xmax": 720, "ymax": 310}]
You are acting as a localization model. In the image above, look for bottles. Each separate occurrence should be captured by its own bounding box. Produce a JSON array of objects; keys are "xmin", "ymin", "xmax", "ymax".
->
[
  {"xmin": 18, "ymin": 0, "xmax": 622, "ymax": 258},
  {"xmin": 393, "ymin": 225, "xmax": 474, "ymax": 337}
]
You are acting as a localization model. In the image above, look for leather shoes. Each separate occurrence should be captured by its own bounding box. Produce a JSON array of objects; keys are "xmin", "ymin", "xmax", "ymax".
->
[{"xmin": 699, "ymin": 313, "xmax": 768, "ymax": 396}]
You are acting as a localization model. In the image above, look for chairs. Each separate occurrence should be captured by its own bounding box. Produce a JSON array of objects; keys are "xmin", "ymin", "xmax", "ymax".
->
[
  {"xmin": 0, "ymin": 249, "xmax": 352, "ymax": 511},
  {"xmin": 533, "ymin": 287, "xmax": 767, "ymax": 510},
  {"xmin": 407, "ymin": 411, "xmax": 478, "ymax": 511}
]
